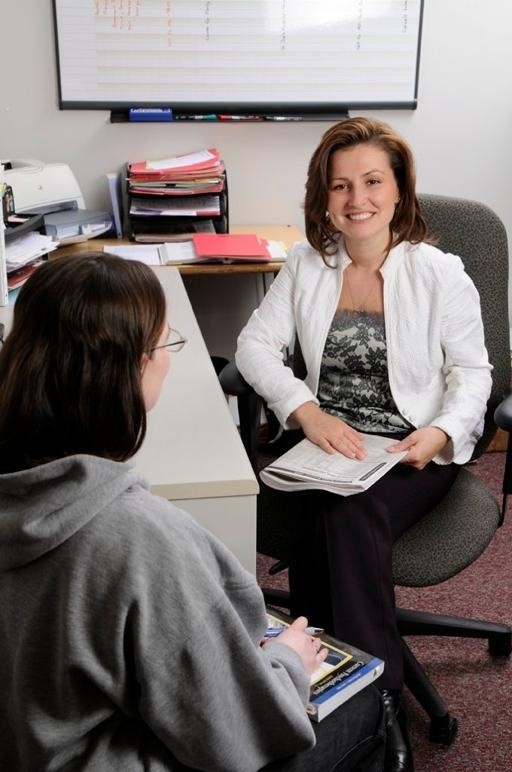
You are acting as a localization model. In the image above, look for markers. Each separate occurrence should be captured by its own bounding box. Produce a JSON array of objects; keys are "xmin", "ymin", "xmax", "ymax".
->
[{"xmin": 173, "ymin": 113, "xmax": 304, "ymax": 122}]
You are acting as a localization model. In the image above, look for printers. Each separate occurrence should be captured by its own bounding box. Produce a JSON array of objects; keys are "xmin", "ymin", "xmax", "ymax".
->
[{"xmin": 0, "ymin": 159, "xmax": 113, "ymax": 275}]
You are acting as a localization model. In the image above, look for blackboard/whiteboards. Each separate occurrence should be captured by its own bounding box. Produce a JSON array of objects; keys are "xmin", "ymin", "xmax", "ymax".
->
[{"xmin": 52, "ymin": 0, "xmax": 423, "ymax": 124}]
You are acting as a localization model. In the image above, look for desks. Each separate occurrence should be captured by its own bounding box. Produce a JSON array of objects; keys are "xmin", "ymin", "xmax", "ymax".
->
[
  {"xmin": 46, "ymin": 225, "xmax": 308, "ymax": 300},
  {"xmin": 2, "ymin": 266, "xmax": 262, "ymax": 580}
]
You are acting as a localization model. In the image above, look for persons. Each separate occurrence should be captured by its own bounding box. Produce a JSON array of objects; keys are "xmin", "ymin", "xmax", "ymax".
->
[
  {"xmin": 0, "ymin": 253, "xmax": 388, "ymax": 772},
  {"xmin": 235, "ymin": 116, "xmax": 495, "ymax": 772}
]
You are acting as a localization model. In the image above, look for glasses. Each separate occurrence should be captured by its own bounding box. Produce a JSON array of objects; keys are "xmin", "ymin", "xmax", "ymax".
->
[{"xmin": 142, "ymin": 328, "xmax": 186, "ymax": 354}]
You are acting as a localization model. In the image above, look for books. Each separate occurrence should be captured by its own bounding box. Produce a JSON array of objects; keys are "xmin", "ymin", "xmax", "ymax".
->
[
  {"xmin": 261, "ymin": 607, "xmax": 386, "ymax": 724},
  {"xmin": 259, "ymin": 432, "xmax": 408, "ymax": 498}
]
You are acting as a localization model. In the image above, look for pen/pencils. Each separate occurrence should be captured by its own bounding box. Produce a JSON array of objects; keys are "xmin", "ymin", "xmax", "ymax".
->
[{"xmin": 263, "ymin": 627, "xmax": 324, "ymax": 637}]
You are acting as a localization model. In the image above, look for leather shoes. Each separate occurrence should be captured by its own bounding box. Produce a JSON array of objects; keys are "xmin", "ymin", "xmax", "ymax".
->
[{"xmin": 378, "ymin": 687, "xmax": 414, "ymax": 772}]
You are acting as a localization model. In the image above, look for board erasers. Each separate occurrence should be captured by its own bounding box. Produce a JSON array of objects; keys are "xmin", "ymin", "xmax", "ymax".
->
[{"xmin": 130, "ymin": 108, "xmax": 172, "ymax": 121}]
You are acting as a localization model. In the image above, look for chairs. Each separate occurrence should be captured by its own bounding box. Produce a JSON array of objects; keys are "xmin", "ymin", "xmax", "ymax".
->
[{"xmin": 217, "ymin": 193, "xmax": 511, "ymax": 660}]
